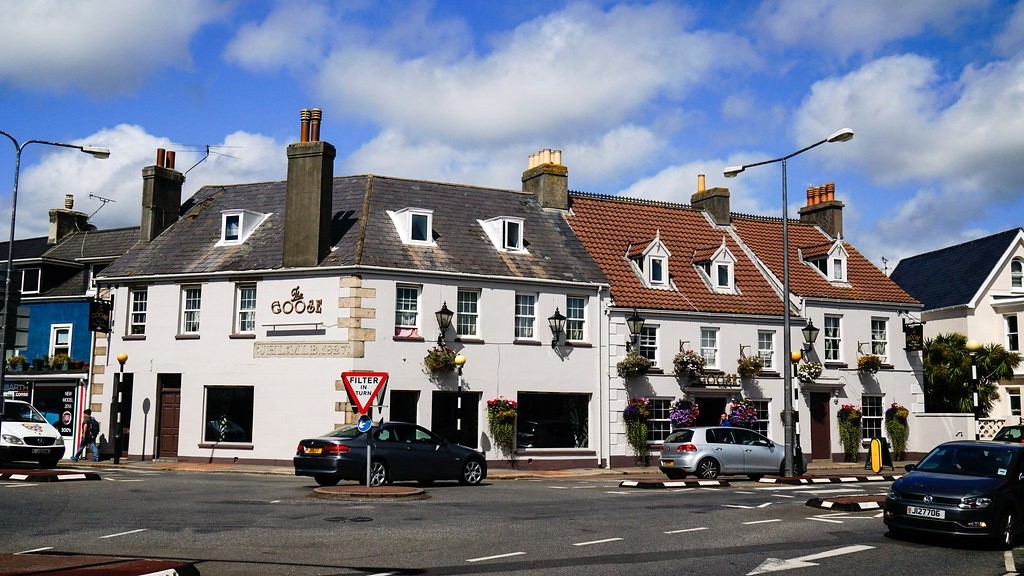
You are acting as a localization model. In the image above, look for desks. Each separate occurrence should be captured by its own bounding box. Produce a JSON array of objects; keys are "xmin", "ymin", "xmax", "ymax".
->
[{"xmin": 32, "ymin": 359, "xmax": 44, "ymax": 371}]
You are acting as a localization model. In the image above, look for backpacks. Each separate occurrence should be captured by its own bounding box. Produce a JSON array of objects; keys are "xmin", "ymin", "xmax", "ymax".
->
[{"xmin": 87, "ymin": 418, "xmax": 99, "ymax": 440}]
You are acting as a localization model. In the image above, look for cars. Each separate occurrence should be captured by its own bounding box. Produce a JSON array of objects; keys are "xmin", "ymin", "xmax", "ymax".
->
[
  {"xmin": 883, "ymin": 440, "xmax": 1024, "ymax": 550},
  {"xmin": 658, "ymin": 426, "xmax": 808, "ymax": 480},
  {"xmin": 293, "ymin": 422, "xmax": 488, "ymax": 487},
  {"xmin": 988, "ymin": 424, "xmax": 1024, "ymax": 444}
]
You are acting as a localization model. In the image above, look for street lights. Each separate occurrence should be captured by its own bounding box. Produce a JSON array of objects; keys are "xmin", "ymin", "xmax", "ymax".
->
[
  {"xmin": 455, "ymin": 355, "xmax": 466, "ymax": 445},
  {"xmin": 114, "ymin": 353, "xmax": 128, "ymax": 464},
  {"xmin": 723, "ymin": 128, "xmax": 854, "ymax": 476},
  {"xmin": 791, "ymin": 351, "xmax": 803, "ymax": 476},
  {"xmin": 0, "ymin": 130, "xmax": 111, "ymax": 417}
]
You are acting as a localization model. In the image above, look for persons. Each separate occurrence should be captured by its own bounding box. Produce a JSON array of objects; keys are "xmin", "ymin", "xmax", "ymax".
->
[
  {"xmin": 70, "ymin": 409, "xmax": 99, "ymax": 462},
  {"xmin": 720, "ymin": 413, "xmax": 730, "ymax": 427}
]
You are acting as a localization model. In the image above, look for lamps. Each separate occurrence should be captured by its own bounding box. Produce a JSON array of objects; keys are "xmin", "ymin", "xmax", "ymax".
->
[
  {"xmin": 625, "ymin": 308, "xmax": 646, "ymax": 352},
  {"xmin": 434, "ymin": 300, "xmax": 455, "ymax": 339},
  {"xmin": 800, "ymin": 316, "xmax": 820, "ymax": 360},
  {"xmin": 547, "ymin": 307, "xmax": 568, "ymax": 350}
]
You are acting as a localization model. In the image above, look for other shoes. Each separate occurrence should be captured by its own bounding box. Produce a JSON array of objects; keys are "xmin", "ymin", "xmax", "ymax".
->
[
  {"xmin": 92, "ymin": 456, "xmax": 99, "ymax": 462},
  {"xmin": 71, "ymin": 457, "xmax": 78, "ymax": 462}
]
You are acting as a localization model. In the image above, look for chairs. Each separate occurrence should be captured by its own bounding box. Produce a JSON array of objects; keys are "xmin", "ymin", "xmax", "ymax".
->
[
  {"xmin": 21, "ymin": 359, "xmax": 30, "ymax": 370},
  {"xmin": 386, "ymin": 430, "xmax": 404, "ymax": 441}
]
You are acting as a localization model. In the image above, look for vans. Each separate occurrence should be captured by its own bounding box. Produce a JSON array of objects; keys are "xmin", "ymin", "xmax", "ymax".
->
[{"xmin": 0, "ymin": 399, "xmax": 65, "ymax": 468}]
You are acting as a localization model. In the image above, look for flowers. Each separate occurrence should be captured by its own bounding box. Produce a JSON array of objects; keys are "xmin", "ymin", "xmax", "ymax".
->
[
  {"xmin": 419, "ymin": 345, "xmax": 456, "ymax": 381},
  {"xmin": 622, "ymin": 397, "xmax": 650, "ymax": 422},
  {"xmin": 839, "ymin": 402, "xmax": 862, "ymax": 413},
  {"xmin": 483, "ymin": 396, "xmax": 518, "ymax": 466},
  {"xmin": 889, "ymin": 402, "xmax": 909, "ymax": 419},
  {"xmin": 857, "ymin": 355, "xmax": 887, "ymax": 380},
  {"xmin": 616, "ymin": 353, "xmax": 654, "ymax": 378},
  {"xmin": 737, "ymin": 353, "xmax": 764, "ymax": 379},
  {"xmin": 797, "ymin": 361, "xmax": 823, "ymax": 383},
  {"xmin": 673, "ymin": 350, "xmax": 706, "ymax": 377}
]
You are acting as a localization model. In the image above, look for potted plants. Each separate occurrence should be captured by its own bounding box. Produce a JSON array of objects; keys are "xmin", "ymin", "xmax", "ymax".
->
[
  {"xmin": 49, "ymin": 354, "xmax": 72, "ymax": 370},
  {"xmin": 669, "ymin": 397, "xmax": 700, "ymax": 427},
  {"xmin": 8, "ymin": 355, "xmax": 27, "ymax": 370},
  {"xmin": 727, "ymin": 399, "xmax": 758, "ymax": 428}
]
